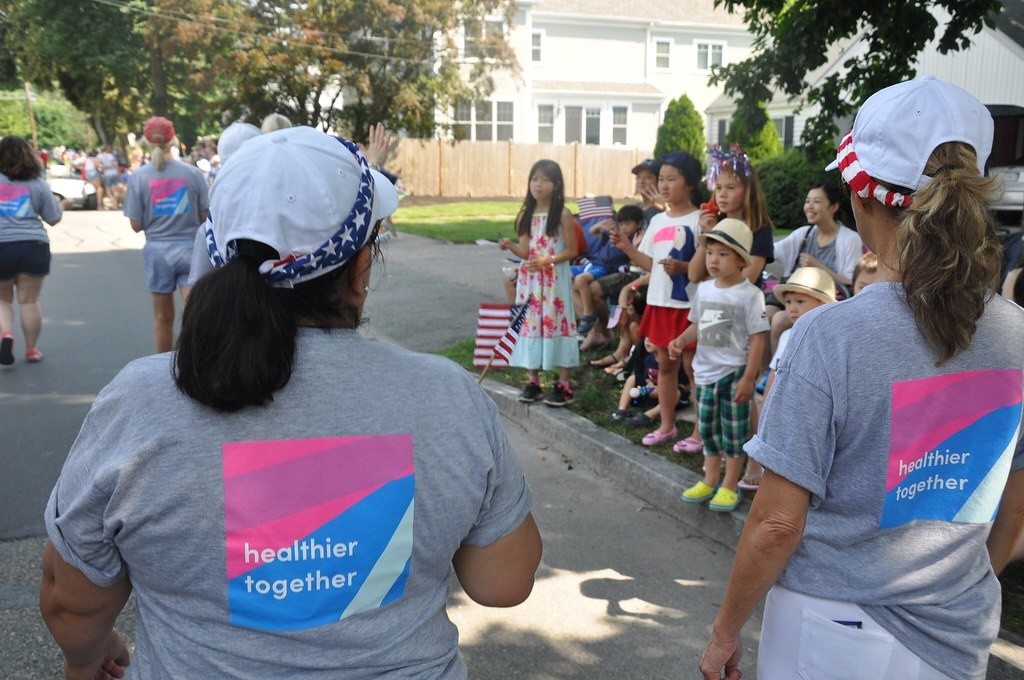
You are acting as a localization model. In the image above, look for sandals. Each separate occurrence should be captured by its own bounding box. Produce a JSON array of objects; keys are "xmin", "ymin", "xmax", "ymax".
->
[
  {"xmin": 642, "ymin": 424, "xmax": 677, "ymax": 445},
  {"xmin": 674, "ymin": 437, "xmax": 703, "ymax": 454}
]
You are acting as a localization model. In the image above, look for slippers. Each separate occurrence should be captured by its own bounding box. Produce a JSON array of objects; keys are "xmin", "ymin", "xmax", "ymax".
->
[
  {"xmin": 709, "ymin": 481, "xmax": 742, "ymax": 512},
  {"xmin": 588, "ymin": 353, "xmax": 627, "ymax": 375},
  {"xmin": 609, "ymin": 409, "xmax": 633, "ymax": 425},
  {"xmin": 577, "ymin": 314, "xmax": 597, "ymax": 333},
  {"xmin": 625, "ymin": 411, "xmax": 658, "ymax": 428},
  {"xmin": 681, "ymin": 480, "xmax": 721, "ymax": 503},
  {"xmin": 736, "ymin": 466, "xmax": 764, "ymax": 490}
]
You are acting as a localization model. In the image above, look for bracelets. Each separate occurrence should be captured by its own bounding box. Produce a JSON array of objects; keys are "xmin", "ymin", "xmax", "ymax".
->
[{"xmin": 550, "ymin": 255, "xmax": 555, "ymax": 267}]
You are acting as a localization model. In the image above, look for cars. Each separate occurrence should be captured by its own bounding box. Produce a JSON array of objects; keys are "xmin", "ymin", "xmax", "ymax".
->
[{"xmin": 46, "ymin": 164, "xmax": 97, "ymax": 210}]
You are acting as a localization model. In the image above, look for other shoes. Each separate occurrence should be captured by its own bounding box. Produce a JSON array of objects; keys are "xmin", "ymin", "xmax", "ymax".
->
[
  {"xmin": 701, "ymin": 456, "xmax": 726, "ymax": 474},
  {"xmin": 26, "ymin": 350, "xmax": 44, "ymax": 361},
  {"xmin": 632, "ymin": 386, "xmax": 656, "ymax": 406},
  {"xmin": 0, "ymin": 331, "xmax": 14, "ymax": 365}
]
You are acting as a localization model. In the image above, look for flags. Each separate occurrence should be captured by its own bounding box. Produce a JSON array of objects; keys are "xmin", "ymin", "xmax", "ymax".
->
[
  {"xmin": 576, "ymin": 195, "xmax": 616, "ymax": 221},
  {"xmin": 492, "ymin": 302, "xmax": 527, "ymax": 367}
]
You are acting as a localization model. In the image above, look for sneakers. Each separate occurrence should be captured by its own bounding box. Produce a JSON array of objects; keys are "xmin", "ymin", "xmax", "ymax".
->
[
  {"xmin": 518, "ymin": 380, "xmax": 544, "ymax": 403},
  {"xmin": 543, "ymin": 381, "xmax": 574, "ymax": 406}
]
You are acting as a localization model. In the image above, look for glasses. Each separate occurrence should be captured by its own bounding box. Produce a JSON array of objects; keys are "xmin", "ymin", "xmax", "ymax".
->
[{"xmin": 840, "ymin": 172, "xmax": 852, "ymax": 200}]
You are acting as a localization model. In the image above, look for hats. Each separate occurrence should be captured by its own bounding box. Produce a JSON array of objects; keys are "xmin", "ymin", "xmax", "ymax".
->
[
  {"xmin": 772, "ymin": 265, "xmax": 839, "ymax": 304},
  {"xmin": 825, "ymin": 76, "xmax": 996, "ymax": 210},
  {"xmin": 204, "ymin": 125, "xmax": 400, "ymax": 288},
  {"xmin": 143, "ymin": 117, "xmax": 174, "ymax": 144},
  {"xmin": 631, "ymin": 159, "xmax": 660, "ymax": 178},
  {"xmin": 661, "ymin": 151, "xmax": 703, "ymax": 202},
  {"xmin": 216, "ymin": 121, "xmax": 263, "ymax": 166},
  {"xmin": 697, "ymin": 218, "xmax": 754, "ymax": 262}
]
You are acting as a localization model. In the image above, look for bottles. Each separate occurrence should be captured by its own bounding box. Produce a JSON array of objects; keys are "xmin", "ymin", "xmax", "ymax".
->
[
  {"xmin": 502, "ymin": 267, "xmax": 518, "ymax": 287},
  {"xmin": 631, "ymin": 284, "xmax": 647, "ymax": 317}
]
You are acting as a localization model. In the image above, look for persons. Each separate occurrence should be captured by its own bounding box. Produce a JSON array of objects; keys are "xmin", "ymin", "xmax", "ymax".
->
[
  {"xmin": 39, "ymin": 131, "xmax": 222, "ymax": 212},
  {"xmin": 0, "ymin": 135, "xmax": 62, "ymax": 365},
  {"xmin": 699, "ymin": 69, "xmax": 1023, "ymax": 680},
  {"xmin": 497, "ymin": 158, "xmax": 580, "ymax": 406},
  {"xmin": 123, "ymin": 116, "xmax": 211, "ymax": 353},
  {"xmin": 261, "ymin": 113, "xmax": 292, "ymax": 134},
  {"xmin": 187, "ymin": 121, "xmax": 264, "ymax": 287},
  {"xmin": 38, "ymin": 125, "xmax": 543, "ymax": 680},
  {"xmin": 505, "ymin": 152, "xmax": 880, "ymax": 514}
]
